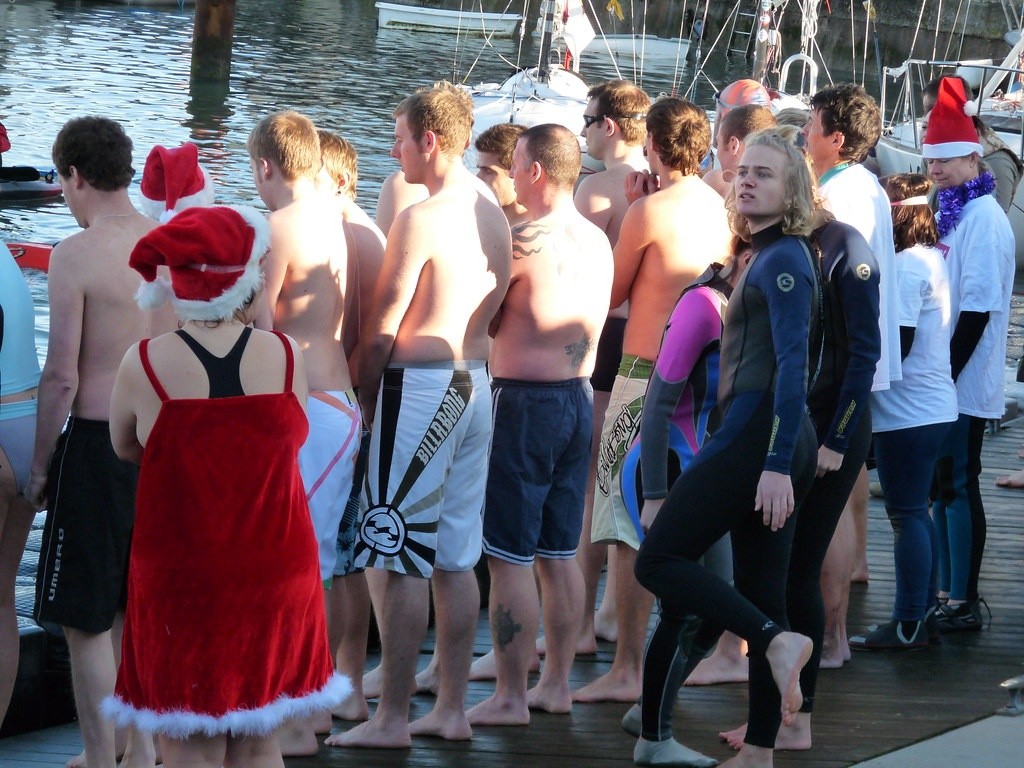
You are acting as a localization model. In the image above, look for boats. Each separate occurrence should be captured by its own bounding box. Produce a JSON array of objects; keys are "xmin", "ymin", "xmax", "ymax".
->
[
  {"xmin": 0, "ymin": 163, "xmax": 62, "ymax": 202},
  {"xmin": 584, "ymin": 32, "xmax": 693, "ymax": 60},
  {"xmin": 374, "ymin": 1, "xmax": 524, "ymax": 38}
]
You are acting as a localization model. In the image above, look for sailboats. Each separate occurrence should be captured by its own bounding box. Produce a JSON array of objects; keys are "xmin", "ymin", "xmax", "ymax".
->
[
  {"xmin": 669, "ymin": 1, "xmax": 1024, "ymax": 276},
  {"xmin": 451, "ymin": 0, "xmax": 647, "ymax": 197}
]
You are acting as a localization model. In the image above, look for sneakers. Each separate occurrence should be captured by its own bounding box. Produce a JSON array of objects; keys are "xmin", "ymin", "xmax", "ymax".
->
[{"xmin": 932, "ymin": 594, "xmax": 992, "ymax": 630}]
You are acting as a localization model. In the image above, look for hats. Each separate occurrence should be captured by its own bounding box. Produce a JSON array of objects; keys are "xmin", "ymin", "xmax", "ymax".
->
[
  {"xmin": 128, "ymin": 203, "xmax": 270, "ymax": 322},
  {"xmin": 921, "ymin": 76, "xmax": 984, "ymax": 158},
  {"xmin": 717, "ymin": 79, "xmax": 770, "ymax": 117},
  {"xmin": 139, "ymin": 145, "xmax": 214, "ymax": 224}
]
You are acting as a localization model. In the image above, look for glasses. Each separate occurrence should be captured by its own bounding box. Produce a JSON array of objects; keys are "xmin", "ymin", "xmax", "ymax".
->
[
  {"xmin": 712, "ymin": 92, "xmax": 740, "ymax": 108},
  {"xmin": 583, "ymin": 114, "xmax": 647, "ymax": 127}
]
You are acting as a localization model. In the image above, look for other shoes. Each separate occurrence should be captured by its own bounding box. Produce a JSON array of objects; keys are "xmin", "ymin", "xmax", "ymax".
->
[{"xmin": 848, "ymin": 619, "xmax": 928, "ymax": 652}]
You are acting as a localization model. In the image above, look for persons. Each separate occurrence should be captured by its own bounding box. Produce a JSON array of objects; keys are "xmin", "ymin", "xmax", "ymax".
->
[
  {"xmin": 619, "ymin": 231, "xmax": 753, "ymax": 767},
  {"xmin": 633, "ymin": 127, "xmax": 814, "ymax": 768},
  {"xmin": 320, "ymin": 79, "xmax": 514, "ymax": 747},
  {"xmin": 101, "ymin": 204, "xmax": 350, "ymax": 768},
  {"xmin": 462, "ymin": 75, "xmax": 1014, "ymax": 748},
  {"xmin": 0, "ymin": 116, "xmax": 387, "ymax": 768}
]
